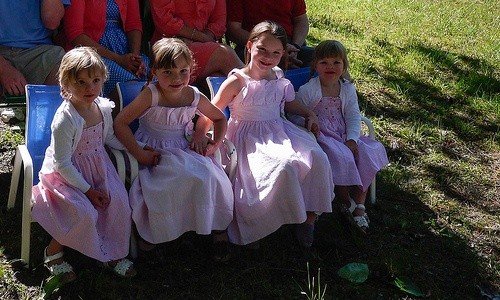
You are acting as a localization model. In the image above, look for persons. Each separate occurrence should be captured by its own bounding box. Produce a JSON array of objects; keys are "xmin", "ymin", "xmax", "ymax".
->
[
  {"xmin": 286, "ymin": 40, "xmax": 389, "ymax": 233},
  {"xmin": 39, "ymin": 46, "xmax": 136, "ymax": 284},
  {"xmin": 65, "ymin": 0, "xmax": 154, "ymax": 118},
  {"xmin": 0, "ymin": 0, "xmax": 66, "ymax": 96},
  {"xmin": 113, "ymin": 37, "xmax": 228, "ymax": 277},
  {"xmin": 191, "ymin": 21, "xmax": 334, "ymax": 258},
  {"xmin": 148, "ymin": 0, "xmax": 246, "ymax": 85},
  {"xmin": 228, "ymin": 0, "xmax": 315, "ymax": 70}
]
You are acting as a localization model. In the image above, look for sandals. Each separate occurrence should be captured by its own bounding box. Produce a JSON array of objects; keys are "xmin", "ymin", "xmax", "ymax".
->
[
  {"xmin": 140, "ymin": 252, "xmax": 159, "ymax": 260},
  {"xmin": 42, "ymin": 246, "xmax": 77, "ymax": 283},
  {"xmin": 296, "ymin": 221, "xmax": 314, "ymax": 248},
  {"xmin": 247, "ymin": 241, "xmax": 262, "ymax": 249},
  {"xmin": 340, "ymin": 198, "xmax": 371, "ymax": 234},
  {"xmin": 210, "ymin": 228, "xmax": 230, "ymax": 261},
  {"xmin": 103, "ymin": 257, "xmax": 136, "ymax": 279}
]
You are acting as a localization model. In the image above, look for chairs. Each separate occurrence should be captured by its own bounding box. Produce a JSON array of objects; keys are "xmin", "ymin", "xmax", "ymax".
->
[
  {"xmin": 284, "ymin": 67, "xmax": 376, "ymax": 204},
  {"xmin": 8, "ymin": 85, "xmax": 125, "ymax": 269},
  {"xmin": 117, "ymin": 81, "xmax": 150, "ymax": 186},
  {"xmin": 206, "ymin": 76, "xmax": 237, "ymax": 182}
]
